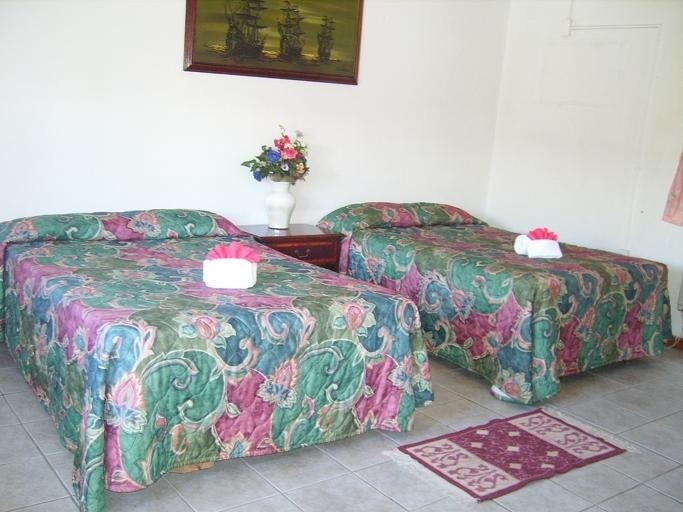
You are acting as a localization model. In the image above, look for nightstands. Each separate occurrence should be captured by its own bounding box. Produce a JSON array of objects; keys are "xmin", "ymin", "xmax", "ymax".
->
[{"xmin": 237, "ymin": 224, "xmax": 341, "ymax": 273}]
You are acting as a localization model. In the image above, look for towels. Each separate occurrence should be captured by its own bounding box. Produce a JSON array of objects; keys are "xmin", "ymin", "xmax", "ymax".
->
[
  {"xmin": 202, "ymin": 241, "xmax": 260, "ymax": 288},
  {"xmin": 514, "ymin": 228, "xmax": 563, "ymax": 259}
]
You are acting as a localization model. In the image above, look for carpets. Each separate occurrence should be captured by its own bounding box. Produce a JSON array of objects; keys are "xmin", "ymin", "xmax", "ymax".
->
[{"xmin": 398, "ymin": 408, "xmax": 625, "ymax": 503}]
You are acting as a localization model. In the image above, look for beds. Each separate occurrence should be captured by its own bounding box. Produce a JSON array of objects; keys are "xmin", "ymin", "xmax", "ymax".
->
[
  {"xmin": 0, "ymin": 209, "xmax": 434, "ymax": 512},
  {"xmin": 318, "ymin": 202, "xmax": 672, "ymax": 405}
]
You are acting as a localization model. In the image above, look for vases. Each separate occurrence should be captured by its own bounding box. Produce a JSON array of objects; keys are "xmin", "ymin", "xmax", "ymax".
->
[{"xmin": 266, "ymin": 182, "xmax": 296, "ymax": 229}]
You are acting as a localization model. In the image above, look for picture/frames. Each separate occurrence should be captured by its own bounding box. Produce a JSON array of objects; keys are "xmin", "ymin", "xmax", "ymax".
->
[{"xmin": 183, "ymin": 0, "xmax": 364, "ymax": 85}]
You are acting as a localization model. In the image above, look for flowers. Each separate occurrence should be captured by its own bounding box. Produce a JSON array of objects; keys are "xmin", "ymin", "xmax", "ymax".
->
[{"xmin": 241, "ymin": 124, "xmax": 310, "ymax": 182}]
